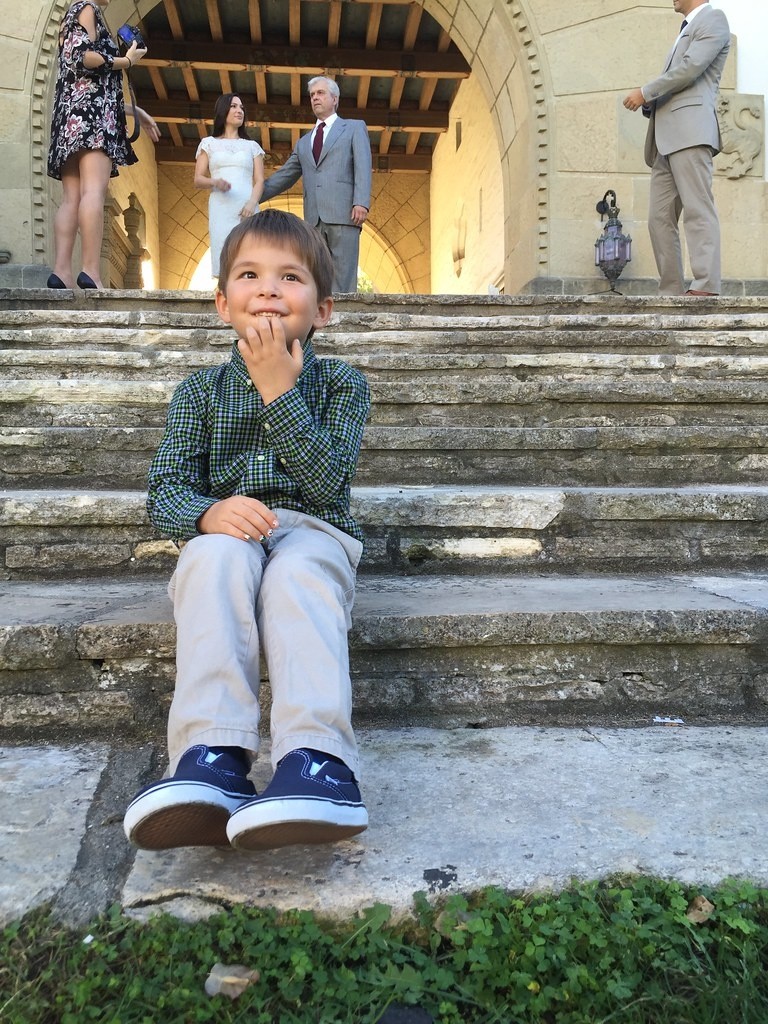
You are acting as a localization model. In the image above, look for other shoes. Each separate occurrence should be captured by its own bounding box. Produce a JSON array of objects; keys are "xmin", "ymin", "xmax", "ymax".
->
[
  {"xmin": 682, "ymin": 290, "xmax": 717, "ymax": 296},
  {"xmin": 77, "ymin": 272, "xmax": 97, "ymax": 289},
  {"xmin": 47, "ymin": 273, "xmax": 67, "ymax": 289},
  {"xmin": 123, "ymin": 744, "xmax": 369, "ymax": 851}
]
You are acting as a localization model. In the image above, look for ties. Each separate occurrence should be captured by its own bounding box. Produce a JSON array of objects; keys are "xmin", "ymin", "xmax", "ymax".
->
[
  {"xmin": 680, "ymin": 20, "xmax": 688, "ymax": 32},
  {"xmin": 312, "ymin": 122, "xmax": 326, "ymax": 166}
]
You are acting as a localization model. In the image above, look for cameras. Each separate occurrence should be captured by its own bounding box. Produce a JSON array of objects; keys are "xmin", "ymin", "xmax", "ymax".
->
[{"xmin": 117, "ymin": 23, "xmax": 145, "ymax": 50}]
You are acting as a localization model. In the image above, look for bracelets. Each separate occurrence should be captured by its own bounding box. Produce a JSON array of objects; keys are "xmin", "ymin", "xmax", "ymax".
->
[{"xmin": 125, "ymin": 56, "xmax": 131, "ymax": 70}]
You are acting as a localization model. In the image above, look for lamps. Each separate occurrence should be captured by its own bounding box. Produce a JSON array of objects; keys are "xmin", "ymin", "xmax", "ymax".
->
[{"xmin": 588, "ymin": 190, "xmax": 632, "ymax": 295}]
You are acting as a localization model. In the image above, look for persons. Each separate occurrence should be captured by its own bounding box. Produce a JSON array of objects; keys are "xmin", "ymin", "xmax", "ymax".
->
[
  {"xmin": 45, "ymin": 0, "xmax": 148, "ymax": 289},
  {"xmin": 622, "ymin": 0, "xmax": 731, "ymax": 296},
  {"xmin": 254, "ymin": 75, "xmax": 371, "ymax": 293},
  {"xmin": 124, "ymin": 206, "xmax": 377, "ymax": 851},
  {"xmin": 192, "ymin": 92, "xmax": 266, "ymax": 291}
]
